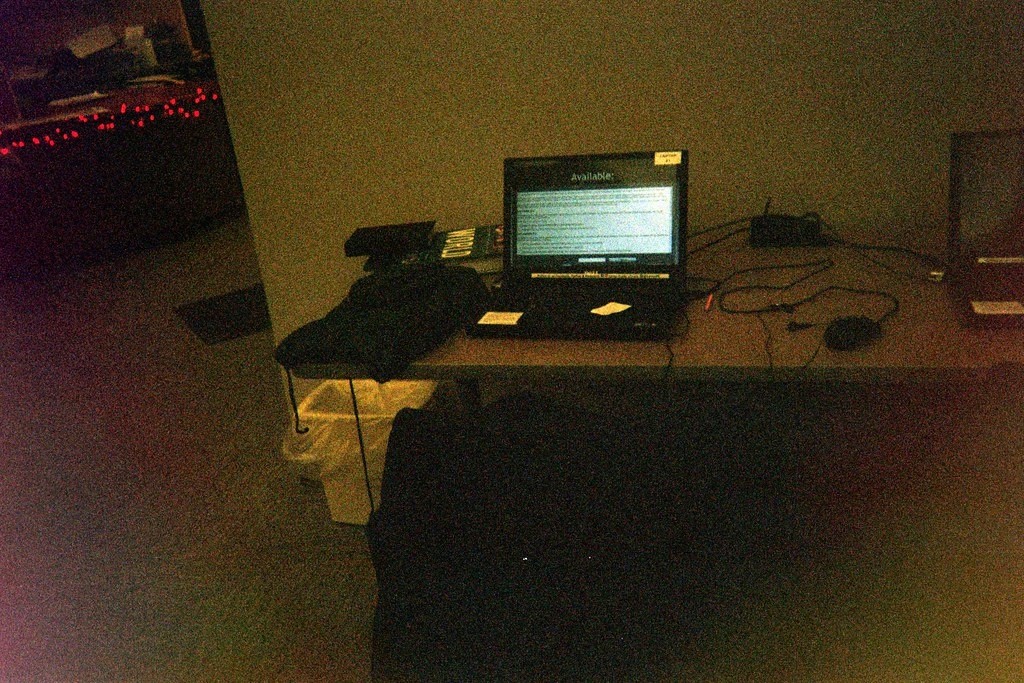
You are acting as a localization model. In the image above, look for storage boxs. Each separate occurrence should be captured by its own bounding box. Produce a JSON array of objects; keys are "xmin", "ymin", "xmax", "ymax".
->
[{"xmin": 9, "ymin": 23, "xmax": 186, "ymax": 120}]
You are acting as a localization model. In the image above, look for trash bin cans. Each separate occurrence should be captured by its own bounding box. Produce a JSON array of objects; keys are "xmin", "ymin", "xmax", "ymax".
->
[{"xmin": 293, "ymin": 380, "xmax": 437, "ymax": 525}]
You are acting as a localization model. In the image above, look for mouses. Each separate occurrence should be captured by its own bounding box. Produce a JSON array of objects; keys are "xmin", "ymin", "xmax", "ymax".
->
[{"xmin": 826, "ymin": 316, "xmax": 880, "ymax": 352}]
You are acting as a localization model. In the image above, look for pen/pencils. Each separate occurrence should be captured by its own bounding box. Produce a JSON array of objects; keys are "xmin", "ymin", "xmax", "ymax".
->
[{"xmin": 706, "ymin": 293, "xmax": 715, "ymax": 311}]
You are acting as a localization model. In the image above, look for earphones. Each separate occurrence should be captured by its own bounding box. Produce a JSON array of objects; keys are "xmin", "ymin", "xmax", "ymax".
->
[
  {"xmin": 786, "ymin": 322, "xmax": 814, "ymax": 331},
  {"xmin": 783, "ymin": 304, "xmax": 793, "ymax": 313}
]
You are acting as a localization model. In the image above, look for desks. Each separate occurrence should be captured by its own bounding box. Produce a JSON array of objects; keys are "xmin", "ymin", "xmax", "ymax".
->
[{"xmin": 291, "ymin": 220, "xmax": 1024, "ymax": 683}]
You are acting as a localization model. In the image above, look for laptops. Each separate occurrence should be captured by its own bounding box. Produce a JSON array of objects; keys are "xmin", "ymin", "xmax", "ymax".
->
[
  {"xmin": 470, "ymin": 149, "xmax": 689, "ymax": 339},
  {"xmin": 946, "ymin": 128, "xmax": 1024, "ymax": 327}
]
